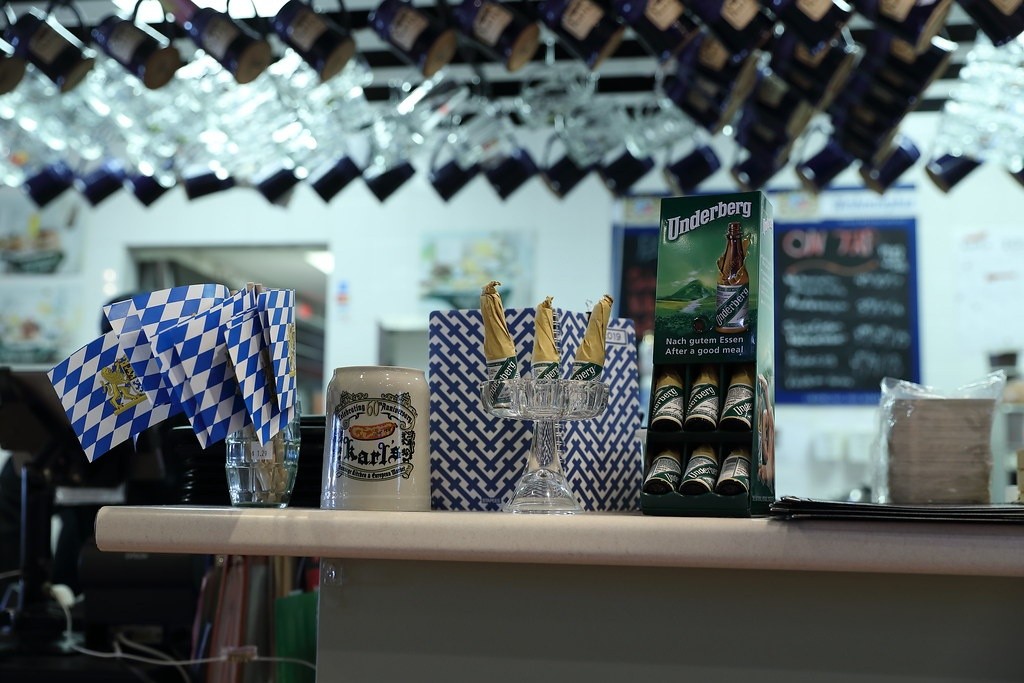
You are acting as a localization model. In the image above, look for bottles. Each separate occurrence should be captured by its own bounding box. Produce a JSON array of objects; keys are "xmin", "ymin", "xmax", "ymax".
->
[
  {"xmin": 642, "ymin": 442, "xmax": 682, "ymax": 494},
  {"xmin": 714, "ymin": 222, "xmax": 749, "ymax": 332},
  {"xmin": 716, "ymin": 440, "xmax": 751, "ymax": 498},
  {"xmin": 720, "ymin": 364, "xmax": 757, "ymax": 430},
  {"xmin": 650, "ymin": 371, "xmax": 684, "ymax": 431},
  {"xmin": 684, "ymin": 367, "xmax": 720, "ymax": 431},
  {"xmin": 679, "ymin": 441, "xmax": 718, "ymax": 494}
]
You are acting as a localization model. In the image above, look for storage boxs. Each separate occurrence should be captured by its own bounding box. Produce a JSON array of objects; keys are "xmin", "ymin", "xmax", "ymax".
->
[
  {"xmin": 430, "ymin": 309, "xmax": 641, "ymax": 514},
  {"xmin": 640, "ymin": 189, "xmax": 777, "ymax": 518}
]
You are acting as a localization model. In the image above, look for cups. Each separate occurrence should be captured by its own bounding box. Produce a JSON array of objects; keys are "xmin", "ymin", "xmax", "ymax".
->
[
  {"xmin": 225, "ymin": 398, "xmax": 303, "ymax": 509},
  {"xmin": 1, "ymin": 1, "xmax": 1024, "ymax": 206}
]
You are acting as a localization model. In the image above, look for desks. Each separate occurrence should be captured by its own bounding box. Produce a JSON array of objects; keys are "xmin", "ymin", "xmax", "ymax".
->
[{"xmin": 95, "ymin": 504, "xmax": 1024, "ymax": 683}]
